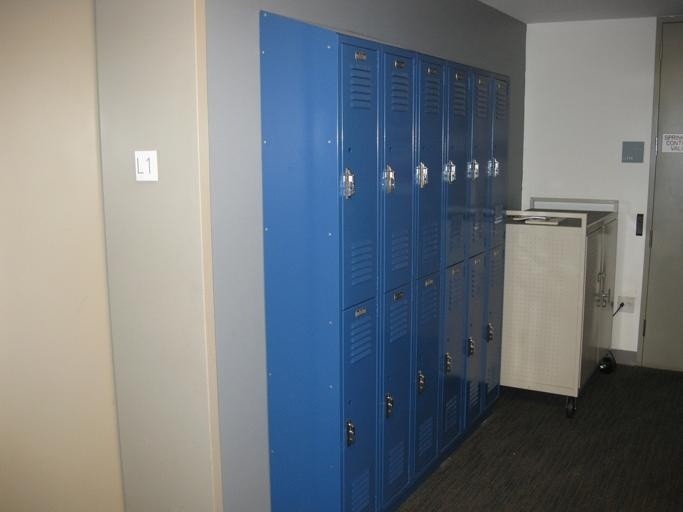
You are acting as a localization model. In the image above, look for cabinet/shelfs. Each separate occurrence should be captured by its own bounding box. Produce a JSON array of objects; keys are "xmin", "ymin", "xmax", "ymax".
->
[{"xmin": 258, "ymin": 8, "xmax": 513, "ymax": 512}]
